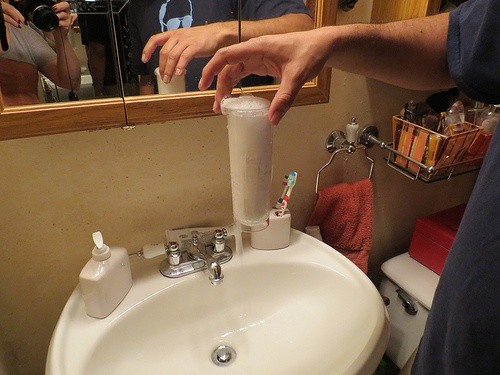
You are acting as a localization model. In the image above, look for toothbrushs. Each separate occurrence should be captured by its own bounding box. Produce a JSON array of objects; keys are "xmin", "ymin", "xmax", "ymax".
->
[{"xmin": 275, "ymin": 171, "xmax": 297, "ymax": 218}]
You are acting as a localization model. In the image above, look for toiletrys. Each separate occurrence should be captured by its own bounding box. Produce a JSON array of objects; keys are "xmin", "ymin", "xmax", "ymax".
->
[{"xmin": 79, "ymin": 230, "xmax": 133, "ymax": 315}]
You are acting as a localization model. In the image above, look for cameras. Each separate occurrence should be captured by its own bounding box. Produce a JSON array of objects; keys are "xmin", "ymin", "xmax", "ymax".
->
[{"xmin": 9, "ymin": 0, "xmax": 60, "ymax": 31}]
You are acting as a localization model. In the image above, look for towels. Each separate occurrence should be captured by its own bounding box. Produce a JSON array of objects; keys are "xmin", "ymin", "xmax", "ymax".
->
[{"xmin": 309, "ymin": 180, "xmax": 374, "ymax": 274}]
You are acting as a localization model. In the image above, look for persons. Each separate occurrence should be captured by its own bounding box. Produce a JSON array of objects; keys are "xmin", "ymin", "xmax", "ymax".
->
[
  {"xmin": 77, "ymin": 1, "xmax": 109, "ymax": 98},
  {"xmin": 127, "ymin": 0, "xmax": 313, "ymax": 96},
  {"xmin": 199, "ymin": 1, "xmax": 500, "ymax": 374},
  {"xmin": 0, "ymin": 0, "xmax": 81, "ymax": 104}
]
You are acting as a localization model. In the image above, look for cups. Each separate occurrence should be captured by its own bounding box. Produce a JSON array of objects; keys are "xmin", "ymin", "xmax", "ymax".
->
[
  {"xmin": 155, "ymin": 69, "xmax": 188, "ymax": 94},
  {"xmin": 223, "ymin": 97, "xmax": 272, "ymax": 233}
]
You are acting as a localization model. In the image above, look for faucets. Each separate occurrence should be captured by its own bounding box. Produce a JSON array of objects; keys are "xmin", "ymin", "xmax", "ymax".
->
[{"xmin": 159, "ymin": 224, "xmax": 238, "ymax": 284}]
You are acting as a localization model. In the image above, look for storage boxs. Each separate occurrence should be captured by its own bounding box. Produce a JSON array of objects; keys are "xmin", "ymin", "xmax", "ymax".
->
[
  {"xmin": 408, "ymin": 205, "xmax": 466, "ymax": 275},
  {"xmin": 390, "ymin": 110, "xmax": 492, "ymax": 181}
]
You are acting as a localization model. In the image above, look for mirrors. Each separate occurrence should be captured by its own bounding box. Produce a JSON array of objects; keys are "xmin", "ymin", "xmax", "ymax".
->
[{"xmin": 0, "ymin": 0, "xmax": 315, "ymax": 108}]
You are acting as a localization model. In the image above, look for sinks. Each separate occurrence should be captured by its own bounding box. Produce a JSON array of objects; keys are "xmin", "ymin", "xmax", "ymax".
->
[{"xmin": 45, "ymin": 230, "xmax": 387, "ymax": 375}]
